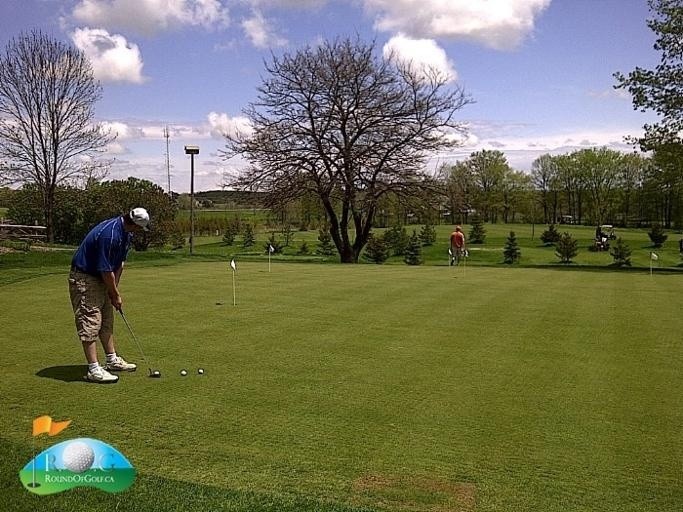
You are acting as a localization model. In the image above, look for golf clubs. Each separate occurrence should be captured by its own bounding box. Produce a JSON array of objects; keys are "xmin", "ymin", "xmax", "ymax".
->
[{"xmin": 119, "ymin": 308, "xmax": 161, "ymax": 377}]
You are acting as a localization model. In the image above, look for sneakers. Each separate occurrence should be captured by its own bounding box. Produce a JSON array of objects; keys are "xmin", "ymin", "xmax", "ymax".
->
[
  {"xmin": 105, "ymin": 356, "xmax": 137, "ymax": 372},
  {"xmin": 86, "ymin": 366, "xmax": 120, "ymax": 384}
]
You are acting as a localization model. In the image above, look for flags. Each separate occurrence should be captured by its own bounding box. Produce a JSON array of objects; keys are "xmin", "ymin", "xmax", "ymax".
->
[
  {"xmin": 229, "ymin": 258, "xmax": 236, "ymax": 271},
  {"xmin": 651, "ymin": 254, "xmax": 658, "ymax": 260},
  {"xmin": 448, "ymin": 247, "xmax": 452, "ymax": 257},
  {"xmin": 463, "ymin": 248, "xmax": 468, "ymax": 257},
  {"xmin": 269, "ymin": 244, "xmax": 274, "ymax": 253}
]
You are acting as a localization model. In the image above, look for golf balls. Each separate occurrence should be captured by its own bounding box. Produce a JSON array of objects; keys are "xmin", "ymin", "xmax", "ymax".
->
[
  {"xmin": 198, "ymin": 368, "xmax": 204, "ymax": 374},
  {"xmin": 154, "ymin": 370, "xmax": 159, "ymax": 374},
  {"xmin": 181, "ymin": 370, "xmax": 186, "ymax": 376}
]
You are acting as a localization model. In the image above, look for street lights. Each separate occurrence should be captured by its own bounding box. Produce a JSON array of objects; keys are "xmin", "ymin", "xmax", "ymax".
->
[{"xmin": 184, "ymin": 146, "xmax": 199, "ymax": 253}]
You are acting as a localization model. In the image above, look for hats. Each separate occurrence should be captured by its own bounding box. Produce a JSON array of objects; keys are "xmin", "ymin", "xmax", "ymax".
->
[
  {"xmin": 129, "ymin": 207, "xmax": 152, "ymax": 232},
  {"xmin": 456, "ymin": 226, "xmax": 461, "ymax": 230}
]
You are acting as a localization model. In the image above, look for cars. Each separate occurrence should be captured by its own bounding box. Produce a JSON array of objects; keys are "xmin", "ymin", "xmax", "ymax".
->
[
  {"xmin": 596, "ymin": 225, "xmax": 616, "ymax": 241},
  {"xmin": 561, "ymin": 215, "xmax": 573, "ymax": 224}
]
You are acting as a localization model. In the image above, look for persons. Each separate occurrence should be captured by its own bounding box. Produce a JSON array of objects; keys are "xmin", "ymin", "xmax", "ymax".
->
[
  {"xmin": 67, "ymin": 206, "xmax": 150, "ymax": 383},
  {"xmin": 450, "ymin": 225, "xmax": 464, "ymax": 266}
]
